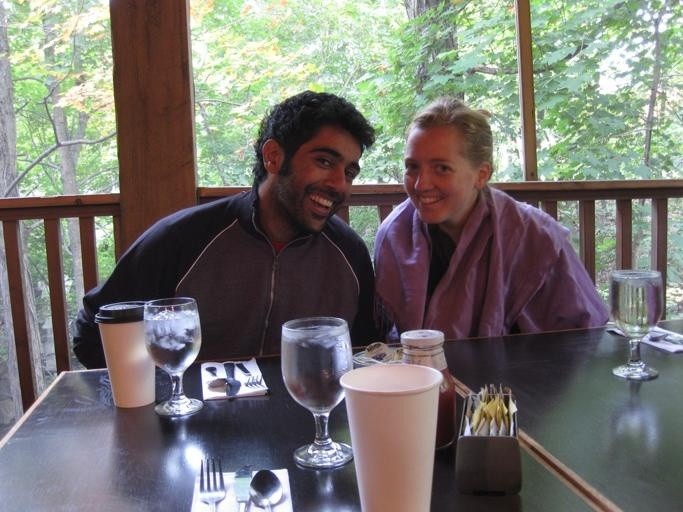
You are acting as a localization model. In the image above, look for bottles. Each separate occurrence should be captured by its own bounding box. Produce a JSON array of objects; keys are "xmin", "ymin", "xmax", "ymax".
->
[{"xmin": 398, "ymin": 328, "xmax": 458, "ymax": 450}]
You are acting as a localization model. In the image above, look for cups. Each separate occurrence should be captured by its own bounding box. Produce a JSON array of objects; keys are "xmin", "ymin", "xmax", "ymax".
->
[
  {"xmin": 93, "ymin": 300, "xmax": 158, "ymax": 409},
  {"xmin": 336, "ymin": 361, "xmax": 445, "ymax": 512}
]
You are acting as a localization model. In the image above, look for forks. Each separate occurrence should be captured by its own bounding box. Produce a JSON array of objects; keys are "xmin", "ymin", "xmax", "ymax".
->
[
  {"xmin": 236, "ymin": 362, "xmax": 263, "ymax": 387},
  {"xmin": 199, "ymin": 456, "xmax": 227, "ymax": 512}
]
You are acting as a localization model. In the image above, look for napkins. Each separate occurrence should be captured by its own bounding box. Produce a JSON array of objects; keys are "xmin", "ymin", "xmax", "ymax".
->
[
  {"xmin": 606, "ymin": 321, "xmax": 683, "ymax": 352},
  {"xmin": 190, "ymin": 469, "xmax": 293, "ymax": 512},
  {"xmin": 200, "ymin": 357, "xmax": 268, "ymax": 400}
]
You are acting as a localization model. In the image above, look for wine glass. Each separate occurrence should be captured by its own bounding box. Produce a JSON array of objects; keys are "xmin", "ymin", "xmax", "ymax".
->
[
  {"xmin": 606, "ymin": 269, "xmax": 664, "ymax": 382},
  {"xmin": 278, "ymin": 315, "xmax": 355, "ymax": 470},
  {"xmin": 142, "ymin": 295, "xmax": 205, "ymax": 418}
]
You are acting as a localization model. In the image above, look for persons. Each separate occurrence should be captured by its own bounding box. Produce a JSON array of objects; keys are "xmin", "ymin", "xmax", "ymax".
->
[
  {"xmin": 374, "ymin": 96, "xmax": 610, "ymax": 340},
  {"xmin": 72, "ymin": 90, "xmax": 375, "ymax": 369}
]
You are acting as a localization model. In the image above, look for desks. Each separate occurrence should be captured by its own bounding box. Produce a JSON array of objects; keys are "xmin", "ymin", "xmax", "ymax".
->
[
  {"xmin": 389, "ymin": 319, "xmax": 683, "ymax": 511},
  {"xmin": 1, "ymin": 345, "xmax": 604, "ymax": 512},
  {"xmin": 1, "ymin": 319, "xmax": 683, "ymax": 512}
]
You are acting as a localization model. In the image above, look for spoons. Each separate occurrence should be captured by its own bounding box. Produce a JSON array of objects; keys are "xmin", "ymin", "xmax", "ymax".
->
[
  {"xmin": 206, "ymin": 366, "xmax": 227, "ymax": 388},
  {"xmin": 248, "ymin": 468, "xmax": 284, "ymax": 512},
  {"xmin": 645, "ymin": 331, "xmax": 669, "ymax": 344}
]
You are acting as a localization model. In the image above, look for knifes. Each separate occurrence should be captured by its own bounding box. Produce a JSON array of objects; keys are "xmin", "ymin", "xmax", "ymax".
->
[
  {"xmin": 223, "ymin": 362, "xmax": 242, "ymax": 397},
  {"xmin": 232, "ymin": 463, "xmax": 253, "ymax": 512}
]
